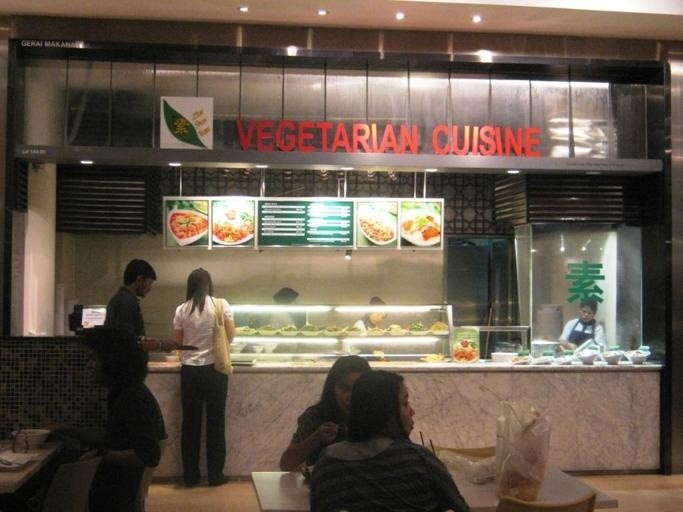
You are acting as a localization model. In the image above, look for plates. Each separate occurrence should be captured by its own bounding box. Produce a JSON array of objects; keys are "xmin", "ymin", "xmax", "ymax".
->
[
  {"xmin": 166, "ymin": 208, "xmax": 208, "ymax": 247},
  {"xmin": 358, "ymin": 205, "xmax": 397, "ymax": 246},
  {"xmin": 401, "ymin": 207, "xmax": 442, "ymax": 247},
  {"xmin": 233, "ymin": 330, "xmax": 450, "ymax": 337},
  {"xmin": 212, "ymin": 233, "xmax": 253, "ymax": 245},
  {"xmin": 453, "ymin": 339, "xmax": 478, "ymax": 363},
  {"xmin": 191, "ymin": 202, "xmax": 208, "ymax": 214},
  {"xmin": 491, "ymin": 352, "xmax": 519, "ymax": 363},
  {"xmin": 513, "ymin": 356, "xmax": 573, "ymax": 365}
]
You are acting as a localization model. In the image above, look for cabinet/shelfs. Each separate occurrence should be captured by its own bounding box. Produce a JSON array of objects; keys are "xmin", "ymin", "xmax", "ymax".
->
[{"xmin": 218, "ymin": 303, "xmax": 454, "ymax": 364}]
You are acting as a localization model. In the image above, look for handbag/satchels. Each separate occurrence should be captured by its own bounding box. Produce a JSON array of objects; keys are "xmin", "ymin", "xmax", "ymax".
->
[{"xmin": 211, "ymin": 297, "xmax": 234, "ymax": 376}]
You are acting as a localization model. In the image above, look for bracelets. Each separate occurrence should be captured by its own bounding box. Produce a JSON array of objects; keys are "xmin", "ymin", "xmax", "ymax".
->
[{"xmin": 152, "ymin": 334, "xmax": 168, "ymax": 355}]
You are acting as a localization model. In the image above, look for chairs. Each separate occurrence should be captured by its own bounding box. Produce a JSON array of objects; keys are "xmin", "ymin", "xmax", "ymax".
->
[
  {"xmin": 500, "ymin": 488, "xmax": 597, "ymax": 510},
  {"xmin": 0, "ymin": 331, "xmax": 157, "ymax": 505},
  {"xmin": 421, "ymin": 442, "xmax": 497, "ymax": 457}
]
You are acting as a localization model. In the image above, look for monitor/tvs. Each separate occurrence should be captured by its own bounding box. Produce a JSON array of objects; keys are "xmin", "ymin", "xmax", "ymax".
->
[{"xmin": 81, "ymin": 305, "xmax": 107, "ymax": 327}]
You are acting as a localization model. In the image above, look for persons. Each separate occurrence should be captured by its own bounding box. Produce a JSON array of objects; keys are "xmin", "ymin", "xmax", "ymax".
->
[
  {"xmin": 172, "ymin": 266, "xmax": 235, "ymax": 487},
  {"xmin": 557, "ymin": 296, "xmax": 605, "ymax": 353},
  {"xmin": 38, "ymin": 324, "xmax": 169, "ymax": 511},
  {"xmin": 265, "ymin": 288, "xmax": 310, "ymax": 327},
  {"xmin": 350, "ymin": 294, "xmax": 391, "ymax": 331},
  {"xmin": 276, "ymin": 354, "xmax": 374, "ymax": 473},
  {"xmin": 306, "ymin": 368, "xmax": 470, "ymax": 511},
  {"xmin": 103, "ymin": 257, "xmax": 181, "ymax": 384}
]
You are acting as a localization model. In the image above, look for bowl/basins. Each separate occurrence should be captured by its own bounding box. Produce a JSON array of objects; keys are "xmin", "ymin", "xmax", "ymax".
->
[
  {"xmin": 252, "ymin": 341, "xmax": 277, "ymax": 352},
  {"xmin": 164, "ymin": 354, "xmax": 178, "ymax": 362},
  {"xmin": 602, "ymin": 352, "xmax": 624, "ymax": 366},
  {"xmin": 12, "ymin": 428, "xmax": 51, "ymax": 450},
  {"xmin": 228, "ymin": 344, "xmax": 248, "ymax": 353},
  {"xmin": 625, "ymin": 348, "xmax": 651, "ymax": 366},
  {"xmin": 575, "ymin": 349, "xmax": 599, "ymax": 365}
]
